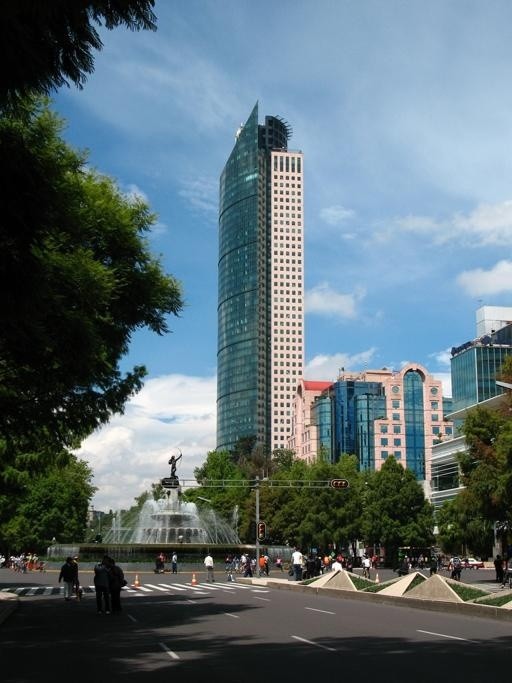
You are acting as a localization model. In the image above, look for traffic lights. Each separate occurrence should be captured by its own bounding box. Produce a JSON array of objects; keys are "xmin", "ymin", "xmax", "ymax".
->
[
  {"xmin": 331, "ymin": 477, "xmax": 350, "ymax": 489},
  {"xmin": 256, "ymin": 520, "xmax": 266, "ymax": 542}
]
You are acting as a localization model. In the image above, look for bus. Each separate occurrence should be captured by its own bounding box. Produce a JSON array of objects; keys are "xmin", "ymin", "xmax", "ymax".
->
[{"xmin": 397, "ymin": 546, "xmax": 433, "ymax": 566}]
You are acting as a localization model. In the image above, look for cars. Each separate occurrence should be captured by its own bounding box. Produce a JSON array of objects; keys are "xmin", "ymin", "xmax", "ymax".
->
[{"xmin": 459, "ymin": 557, "xmax": 485, "ymax": 569}]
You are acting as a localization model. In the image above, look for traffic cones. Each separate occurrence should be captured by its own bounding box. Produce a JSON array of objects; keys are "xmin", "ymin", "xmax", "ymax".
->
[
  {"xmin": 133, "ymin": 573, "xmax": 141, "ymax": 587},
  {"xmin": 375, "ymin": 570, "xmax": 379, "ymax": 584},
  {"xmin": 191, "ymin": 573, "xmax": 199, "ymax": 585}
]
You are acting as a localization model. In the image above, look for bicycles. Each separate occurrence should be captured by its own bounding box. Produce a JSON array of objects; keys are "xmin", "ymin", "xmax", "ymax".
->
[
  {"xmin": 361, "ymin": 566, "xmax": 371, "ymax": 579},
  {"xmin": 258, "ymin": 564, "xmax": 271, "ymax": 576}
]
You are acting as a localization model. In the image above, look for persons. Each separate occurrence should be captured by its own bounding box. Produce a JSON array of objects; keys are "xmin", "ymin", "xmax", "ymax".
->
[
  {"xmin": 291, "ymin": 546, "xmax": 378, "ymax": 581},
  {"xmin": 71, "ymin": 556, "xmax": 79, "ymax": 594},
  {"xmin": 393, "ymin": 552, "xmax": 471, "ymax": 582},
  {"xmin": 58, "ymin": 557, "xmax": 77, "ymax": 600},
  {"xmin": 500, "ymin": 557, "xmax": 507, "ymax": 578},
  {"xmin": 107, "ymin": 558, "xmax": 124, "ymax": 611},
  {"xmin": 170, "ymin": 551, "xmax": 178, "ymax": 574},
  {"xmin": 493, "ymin": 554, "xmax": 504, "ymax": 582},
  {"xmin": 205, "ymin": 552, "xmax": 215, "ymax": 581},
  {"xmin": 8, "ymin": 550, "xmax": 38, "ymax": 575},
  {"xmin": 155, "ymin": 553, "xmax": 162, "ymax": 573},
  {"xmin": 93, "ymin": 557, "xmax": 111, "ymax": 614},
  {"xmin": 224, "ymin": 549, "xmax": 268, "ymax": 577},
  {"xmin": 168, "ymin": 452, "xmax": 182, "ymax": 476},
  {"xmin": 499, "ymin": 556, "xmax": 512, "ymax": 588},
  {"xmin": 160, "ymin": 552, "xmax": 166, "ymax": 570},
  {"xmin": 276, "ymin": 555, "xmax": 284, "ymax": 572}
]
